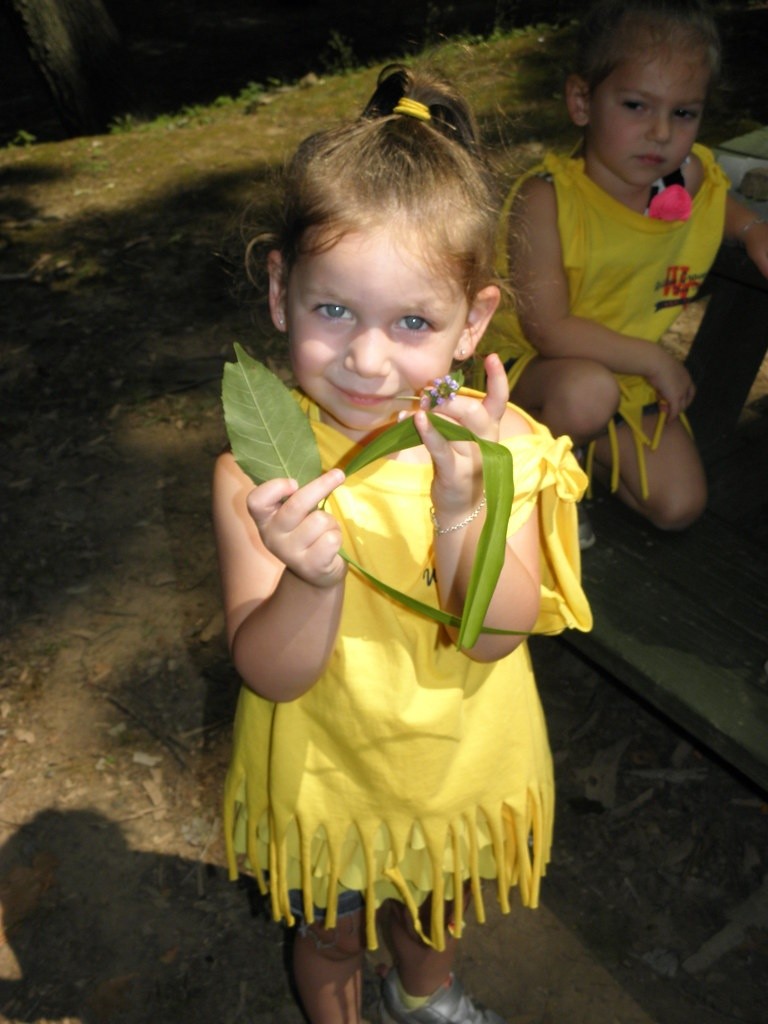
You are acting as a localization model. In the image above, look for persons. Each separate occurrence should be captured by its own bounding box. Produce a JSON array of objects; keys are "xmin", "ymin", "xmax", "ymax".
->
[
  {"xmin": 212, "ymin": 61, "xmax": 593, "ymax": 1022},
  {"xmin": 464, "ymin": 0, "xmax": 768, "ymax": 551}
]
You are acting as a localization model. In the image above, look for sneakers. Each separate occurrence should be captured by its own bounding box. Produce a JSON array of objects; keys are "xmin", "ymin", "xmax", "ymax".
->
[{"xmin": 375, "ymin": 963, "xmax": 505, "ymax": 1024}]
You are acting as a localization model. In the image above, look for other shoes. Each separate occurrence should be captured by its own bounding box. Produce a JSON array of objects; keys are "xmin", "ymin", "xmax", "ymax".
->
[{"xmin": 577, "ymin": 505, "xmax": 595, "ymax": 548}]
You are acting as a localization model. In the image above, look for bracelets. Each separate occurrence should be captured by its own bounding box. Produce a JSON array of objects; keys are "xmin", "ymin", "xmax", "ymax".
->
[
  {"xmin": 739, "ymin": 219, "xmax": 763, "ymax": 245},
  {"xmin": 430, "ymin": 499, "xmax": 485, "ymax": 533}
]
